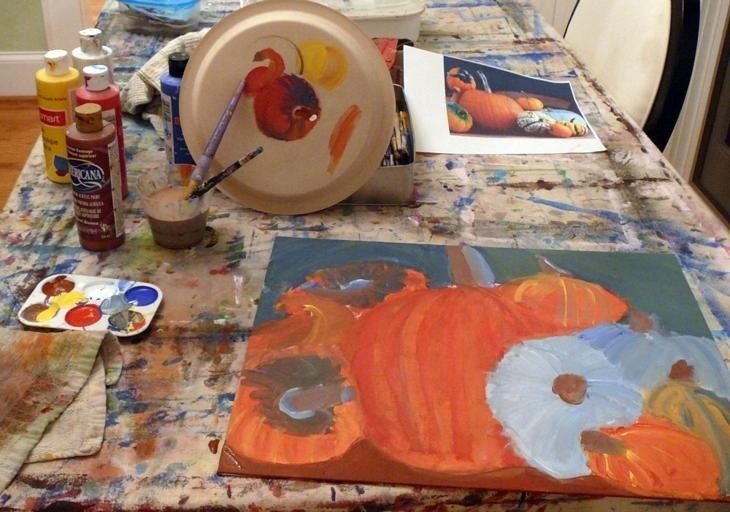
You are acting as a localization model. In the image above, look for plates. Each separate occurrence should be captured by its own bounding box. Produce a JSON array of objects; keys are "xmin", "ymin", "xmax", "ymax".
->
[{"xmin": 179, "ymin": 0, "xmax": 396, "ymax": 218}]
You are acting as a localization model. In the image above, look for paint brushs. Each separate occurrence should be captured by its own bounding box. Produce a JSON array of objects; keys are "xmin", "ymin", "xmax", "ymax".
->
[
  {"xmin": 382, "ymin": 101, "xmax": 413, "ymax": 167},
  {"xmin": 183, "ymin": 79, "xmax": 263, "ymax": 203}
]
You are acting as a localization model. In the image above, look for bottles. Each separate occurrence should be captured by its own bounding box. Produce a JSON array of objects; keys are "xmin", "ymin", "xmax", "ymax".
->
[
  {"xmin": 37, "ymin": 29, "xmax": 128, "ymax": 252},
  {"xmin": 158, "ymin": 52, "xmax": 196, "ymax": 166}
]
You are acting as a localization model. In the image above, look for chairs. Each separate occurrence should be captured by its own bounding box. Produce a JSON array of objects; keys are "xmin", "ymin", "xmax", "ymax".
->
[{"xmin": 560, "ymin": 2, "xmax": 702, "ymax": 157}]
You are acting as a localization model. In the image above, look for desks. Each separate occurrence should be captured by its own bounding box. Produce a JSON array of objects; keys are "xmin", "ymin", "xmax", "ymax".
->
[{"xmin": 1, "ymin": 1, "xmax": 730, "ymax": 512}]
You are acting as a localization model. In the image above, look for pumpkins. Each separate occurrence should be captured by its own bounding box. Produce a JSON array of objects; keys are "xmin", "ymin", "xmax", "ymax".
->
[
  {"xmin": 255, "ymin": 75, "xmax": 321, "ymax": 139},
  {"xmin": 447, "ymin": 68, "xmax": 587, "ymax": 137}
]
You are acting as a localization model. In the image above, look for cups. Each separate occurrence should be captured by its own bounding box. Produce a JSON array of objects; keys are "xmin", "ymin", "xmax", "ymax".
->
[{"xmin": 138, "ymin": 165, "xmax": 215, "ymax": 249}]
